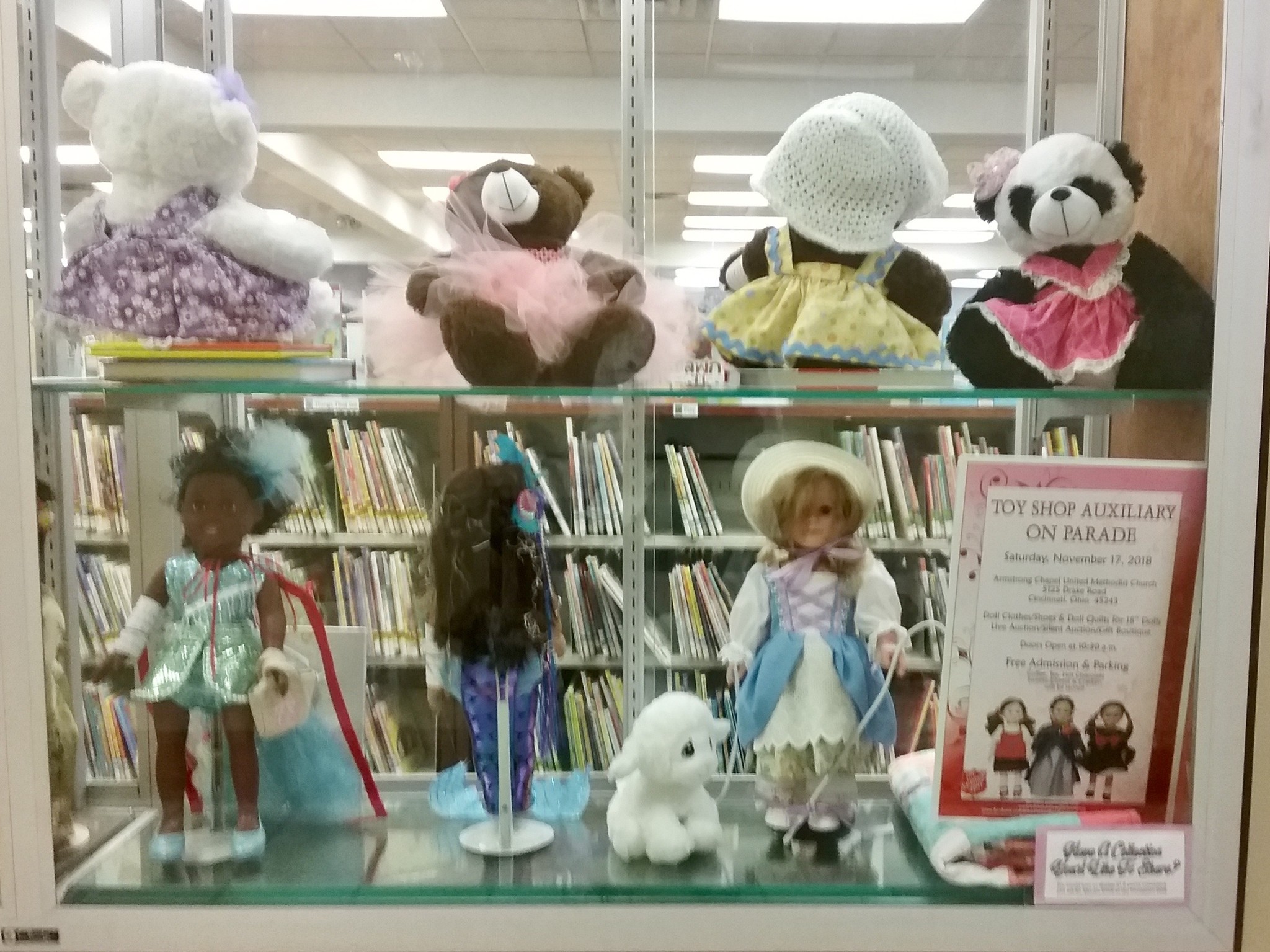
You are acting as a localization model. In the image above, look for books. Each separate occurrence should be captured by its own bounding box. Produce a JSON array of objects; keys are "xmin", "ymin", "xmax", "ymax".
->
[{"xmin": 68, "ymin": 416, "xmax": 1094, "ymax": 783}]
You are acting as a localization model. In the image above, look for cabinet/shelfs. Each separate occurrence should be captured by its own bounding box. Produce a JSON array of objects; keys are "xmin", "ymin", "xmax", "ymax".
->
[{"xmin": 15, "ymin": 0, "xmax": 1127, "ymax": 909}]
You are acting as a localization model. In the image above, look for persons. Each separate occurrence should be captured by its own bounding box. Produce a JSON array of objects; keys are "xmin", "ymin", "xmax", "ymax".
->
[
  {"xmin": 716, "ymin": 440, "xmax": 916, "ymax": 858},
  {"xmin": 1023, "ymin": 696, "xmax": 1086, "ymax": 795},
  {"xmin": 984, "ymin": 697, "xmax": 1035, "ymax": 799},
  {"xmin": 1082, "ymin": 700, "xmax": 1136, "ymax": 798},
  {"xmin": 422, "ymin": 461, "xmax": 567, "ymax": 816},
  {"xmin": 92, "ymin": 433, "xmax": 292, "ymax": 866}
]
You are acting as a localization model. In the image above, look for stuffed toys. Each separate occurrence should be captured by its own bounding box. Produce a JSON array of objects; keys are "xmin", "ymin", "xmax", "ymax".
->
[
  {"xmin": 58, "ymin": 58, "xmax": 340, "ymax": 348},
  {"xmin": 945, "ymin": 133, "xmax": 1214, "ymax": 390},
  {"xmin": 701, "ymin": 93, "xmax": 952, "ymax": 369},
  {"xmin": 605, "ymin": 690, "xmax": 732, "ymax": 865},
  {"xmin": 401, "ymin": 161, "xmax": 656, "ymax": 388}
]
